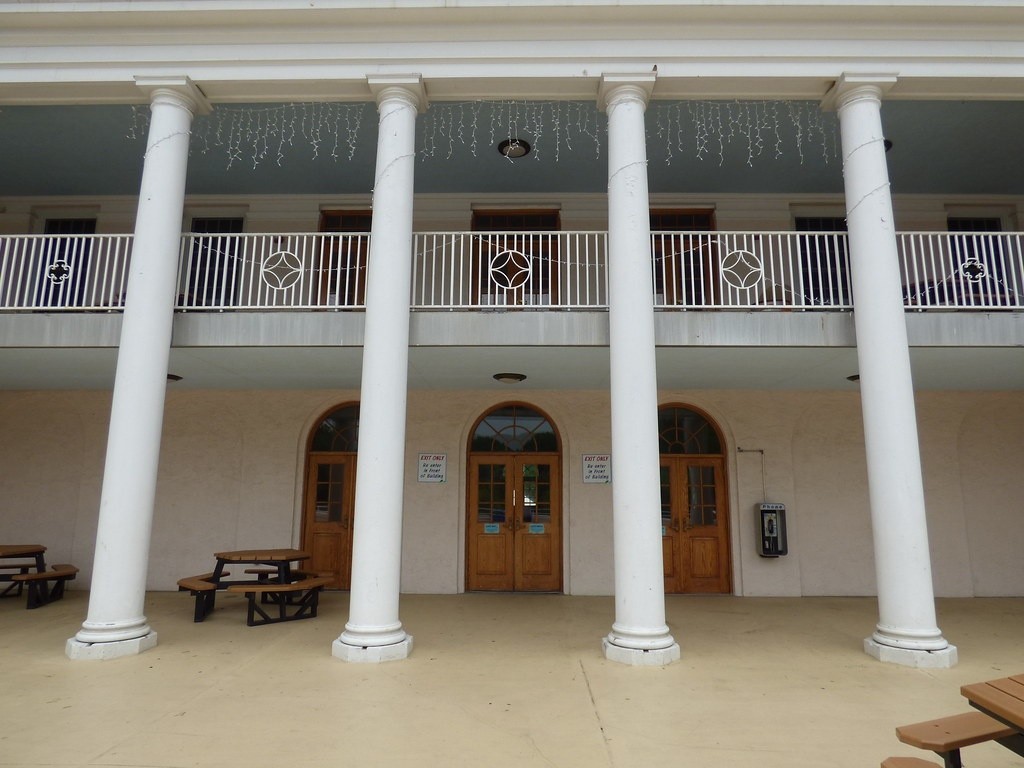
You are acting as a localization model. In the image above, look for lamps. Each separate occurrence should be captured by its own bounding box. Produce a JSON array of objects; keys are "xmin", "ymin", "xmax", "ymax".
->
[
  {"xmin": 493, "ymin": 373, "xmax": 526, "ymax": 384},
  {"xmin": 168, "ymin": 374, "xmax": 182, "ymax": 384},
  {"xmin": 884, "ymin": 137, "xmax": 892, "ymax": 154},
  {"xmin": 499, "ymin": 139, "xmax": 530, "ymax": 158},
  {"xmin": 847, "ymin": 375, "xmax": 861, "ymax": 382}
]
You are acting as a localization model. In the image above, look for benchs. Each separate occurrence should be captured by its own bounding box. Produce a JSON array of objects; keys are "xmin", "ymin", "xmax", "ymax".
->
[
  {"xmin": 227, "ymin": 578, "xmax": 334, "ymax": 627},
  {"xmin": 177, "ymin": 571, "xmax": 229, "ymax": 622},
  {"xmin": 0, "ymin": 564, "xmax": 45, "ymax": 597},
  {"xmin": 12, "ymin": 564, "xmax": 78, "ymax": 610},
  {"xmin": 883, "ymin": 757, "xmax": 943, "ymax": 767},
  {"xmin": 245, "ymin": 569, "xmax": 334, "ymax": 606},
  {"xmin": 896, "ymin": 712, "xmax": 1022, "ymax": 768}
]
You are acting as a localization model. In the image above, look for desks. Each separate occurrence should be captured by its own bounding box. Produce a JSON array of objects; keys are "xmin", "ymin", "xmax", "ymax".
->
[
  {"xmin": 0, "ymin": 545, "xmax": 47, "ymax": 597},
  {"xmin": 961, "ymin": 672, "xmax": 1024, "ymax": 757},
  {"xmin": 206, "ymin": 550, "xmax": 312, "ymax": 613}
]
